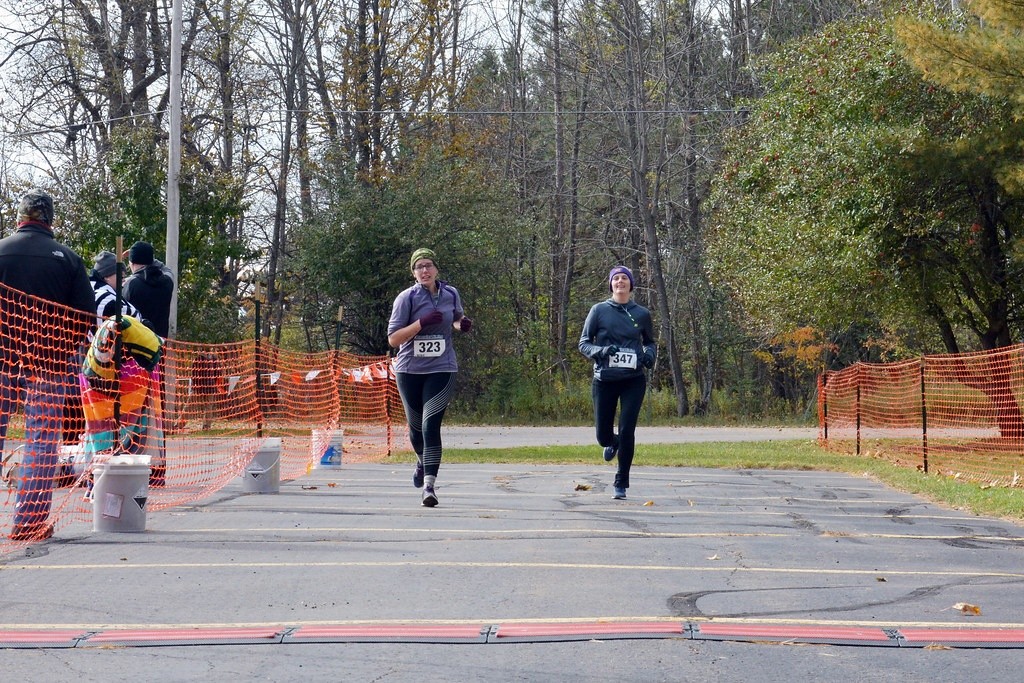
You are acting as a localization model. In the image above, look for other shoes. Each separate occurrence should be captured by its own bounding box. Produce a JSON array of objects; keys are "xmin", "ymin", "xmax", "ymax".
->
[
  {"xmin": 83, "ymin": 478, "xmax": 95, "ymax": 500},
  {"xmin": 10, "ymin": 525, "xmax": 54, "ymax": 541},
  {"xmin": 147, "ymin": 466, "xmax": 167, "ymax": 486}
]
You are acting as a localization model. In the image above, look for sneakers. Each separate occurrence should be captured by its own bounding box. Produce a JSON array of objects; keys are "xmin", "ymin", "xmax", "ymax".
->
[
  {"xmin": 611, "ymin": 486, "xmax": 627, "ymax": 499},
  {"xmin": 421, "ymin": 487, "xmax": 439, "ymax": 507},
  {"xmin": 413, "ymin": 461, "xmax": 425, "ymax": 488},
  {"xmin": 603, "ymin": 433, "xmax": 619, "ymax": 461}
]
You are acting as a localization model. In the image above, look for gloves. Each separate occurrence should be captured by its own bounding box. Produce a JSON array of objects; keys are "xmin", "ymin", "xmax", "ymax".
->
[
  {"xmin": 153, "ymin": 258, "xmax": 163, "ymax": 268},
  {"xmin": 460, "ymin": 316, "xmax": 473, "ymax": 333},
  {"xmin": 602, "ymin": 344, "xmax": 620, "ymax": 358},
  {"xmin": 638, "ymin": 350, "xmax": 654, "ymax": 369},
  {"xmin": 419, "ymin": 311, "xmax": 443, "ymax": 328}
]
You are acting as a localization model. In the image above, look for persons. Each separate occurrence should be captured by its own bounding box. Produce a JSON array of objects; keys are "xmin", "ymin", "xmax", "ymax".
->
[
  {"xmin": 578, "ymin": 265, "xmax": 656, "ymax": 499},
  {"xmin": 60, "ymin": 238, "xmax": 174, "ymax": 488},
  {"xmin": 386, "ymin": 248, "xmax": 473, "ymax": 507},
  {"xmin": 0, "ymin": 188, "xmax": 96, "ymax": 541},
  {"xmin": 174, "ymin": 344, "xmax": 222, "ymax": 430}
]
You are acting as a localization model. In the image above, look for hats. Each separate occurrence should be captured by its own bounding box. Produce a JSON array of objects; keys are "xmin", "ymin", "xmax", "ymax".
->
[
  {"xmin": 94, "ymin": 251, "xmax": 126, "ymax": 278},
  {"xmin": 609, "ymin": 266, "xmax": 634, "ymax": 292},
  {"xmin": 410, "ymin": 247, "xmax": 439, "ymax": 272},
  {"xmin": 129, "ymin": 241, "xmax": 154, "ymax": 265},
  {"xmin": 17, "ymin": 188, "xmax": 53, "ymax": 225}
]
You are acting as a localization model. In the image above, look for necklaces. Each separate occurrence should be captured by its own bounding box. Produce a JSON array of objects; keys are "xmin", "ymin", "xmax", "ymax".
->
[{"xmin": 621, "ymin": 305, "xmax": 639, "ymax": 328}]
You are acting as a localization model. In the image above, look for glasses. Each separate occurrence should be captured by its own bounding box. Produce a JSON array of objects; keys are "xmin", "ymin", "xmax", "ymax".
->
[{"xmin": 412, "ymin": 263, "xmax": 435, "ymax": 271}]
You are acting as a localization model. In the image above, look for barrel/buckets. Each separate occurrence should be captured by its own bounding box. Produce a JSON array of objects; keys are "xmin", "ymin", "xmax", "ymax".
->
[
  {"xmin": 238, "ymin": 438, "xmax": 282, "ymax": 494},
  {"xmin": 311, "ymin": 430, "xmax": 345, "ymax": 470},
  {"xmin": 92, "ymin": 454, "xmax": 153, "ymax": 532}
]
